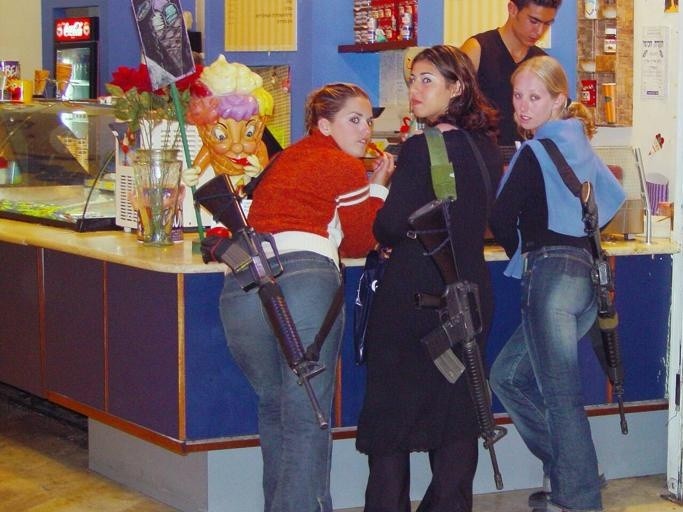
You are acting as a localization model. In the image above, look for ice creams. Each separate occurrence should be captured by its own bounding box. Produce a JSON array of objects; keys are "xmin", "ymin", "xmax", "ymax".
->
[{"xmin": 648, "ymin": 133, "xmax": 664, "ymax": 155}]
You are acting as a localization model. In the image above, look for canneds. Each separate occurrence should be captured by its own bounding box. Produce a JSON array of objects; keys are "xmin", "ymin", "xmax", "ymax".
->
[
  {"xmin": 401, "ymin": 13, "xmax": 412, "ymax": 41},
  {"xmin": 367, "ymin": 18, "xmax": 377, "ymax": 44}
]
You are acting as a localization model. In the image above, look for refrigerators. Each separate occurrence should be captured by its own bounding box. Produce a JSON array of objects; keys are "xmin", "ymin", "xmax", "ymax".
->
[{"xmin": 52, "ymin": 19, "xmax": 97, "ymax": 99}]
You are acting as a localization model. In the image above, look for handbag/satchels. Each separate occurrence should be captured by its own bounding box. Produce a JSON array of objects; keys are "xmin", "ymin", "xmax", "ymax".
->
[{"xmin": 353, "ymin": 242, "xmax": 389, "ymax": 367}]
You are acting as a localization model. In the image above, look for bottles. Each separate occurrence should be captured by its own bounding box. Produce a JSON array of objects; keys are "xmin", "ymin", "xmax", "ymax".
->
[
  {"xmin": 66, "ymin": 63, "xmax": 89, "ymax": 83},
  {"xmin": 365, "ymin": 2, "xmax": 414, "ymax": 42},
  {"xmin": 577, "ymin": 0, "xmax": 618, "ymax": 52},
  {"xmin": 580, "ymin": 80, "xmax": 596, "ymax": 108}
]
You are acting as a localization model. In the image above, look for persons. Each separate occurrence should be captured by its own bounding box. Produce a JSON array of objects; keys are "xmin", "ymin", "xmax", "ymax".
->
[
  {"xmin": 354, "ymin": 41, "xmax": 517, "ymax": 512},
  {"xmin": 179, "ymin": 51, "xmax": 270, "ymax": 193},
  {"xmin": 487, "ymin": 56, "xmax": 627, "ymax": 512},
  {"xmin": 459, "ymin": 0, "xmax": 562, "ymax": 149},
  {"xmin": 219, "ymin": 80, "xmax": 394, "ymax": 511}
]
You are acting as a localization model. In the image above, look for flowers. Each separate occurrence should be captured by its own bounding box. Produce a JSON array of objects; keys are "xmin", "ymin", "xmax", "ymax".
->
[{"xmin": 108, "ymin": 64, "xmax": 210, "ymax": 229}]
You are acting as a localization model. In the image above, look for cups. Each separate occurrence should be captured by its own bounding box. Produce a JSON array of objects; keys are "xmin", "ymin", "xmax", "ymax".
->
[{"xmin": 602, "ymin": 81, "xmax": 617, "ymax": 124}]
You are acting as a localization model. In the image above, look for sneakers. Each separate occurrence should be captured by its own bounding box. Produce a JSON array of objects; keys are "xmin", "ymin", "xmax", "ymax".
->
[{"xmin": 529, "ymin": 490, "xmax": 564, "ymax": 512}]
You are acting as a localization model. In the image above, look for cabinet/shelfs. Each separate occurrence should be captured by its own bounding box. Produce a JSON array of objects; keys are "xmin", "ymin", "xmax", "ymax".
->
[
  {"xmin": 575, "ymin": 0, "xmax": 633, "ymax": 126},
  {"xmin": 0, "ymin": 98, "xmax": 119, "ymax": 233}
]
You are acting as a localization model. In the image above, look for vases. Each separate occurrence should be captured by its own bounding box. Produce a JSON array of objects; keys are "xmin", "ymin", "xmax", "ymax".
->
[{"xmin": 130, "ymin": 149, "xmax": 183, "ymax": 246}]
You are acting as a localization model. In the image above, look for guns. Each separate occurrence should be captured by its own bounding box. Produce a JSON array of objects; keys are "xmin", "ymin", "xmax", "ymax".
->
[
  {"xmin": 408, "ymin": 199, "xmax": 507, "ymax": 490},
  {"xmin": 582, "ymin": 181, "xmax": 627, "ymax": 434},
  {"xmin": 193, "ymin": 174, "xmax": 328, "ymax": 430}
]
